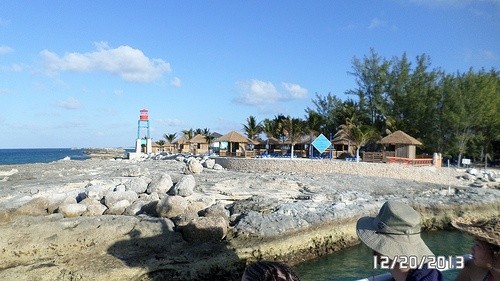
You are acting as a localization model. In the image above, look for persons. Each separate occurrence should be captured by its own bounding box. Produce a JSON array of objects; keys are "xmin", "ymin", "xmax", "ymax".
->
[
  {"xmin": 450, "ymin": 213, "xmax": 500, "ymax": 280},
  {"xmin": 240, "ymin": 261, "xmax": 301, "ymax": 281},
  {"xmin": 356, "ymin": 200, "xmax": 444, "ymax": 280}
]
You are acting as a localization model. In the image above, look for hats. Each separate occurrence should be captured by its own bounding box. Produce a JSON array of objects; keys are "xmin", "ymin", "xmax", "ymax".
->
[
  {"xmin": 450, "ymin": 213, "xmax": 499, "ymax": 246},
  {"xmin": 356, "ymin": 201, "xmax": 435, "ymax": 263}
]
